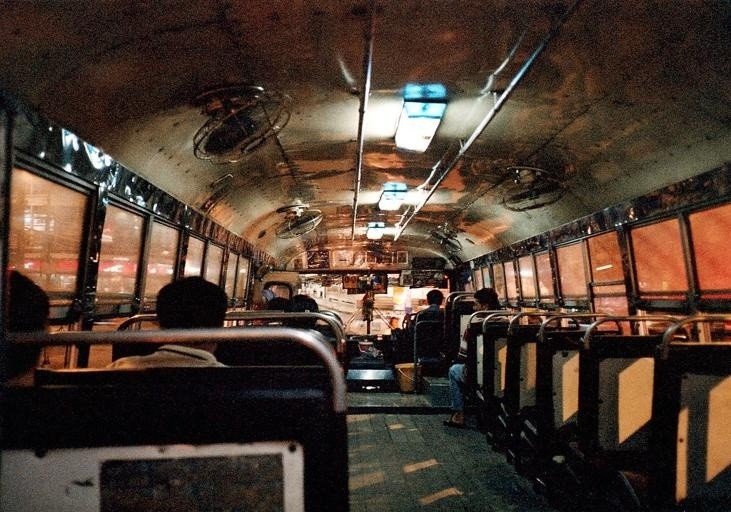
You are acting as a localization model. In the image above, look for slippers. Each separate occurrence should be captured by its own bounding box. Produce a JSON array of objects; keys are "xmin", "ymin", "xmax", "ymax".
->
[{"xmin": 443, "ymin": 418, "xmax": 466, "ymax": 427}]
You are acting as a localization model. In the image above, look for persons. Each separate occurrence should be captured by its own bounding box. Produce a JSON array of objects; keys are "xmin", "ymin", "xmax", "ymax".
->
[
  {"xmin": 409, "ymin": 287, "xmax": 445, "ymax": 328},
  {"xmin": 442, "ymin": 288, "xmax": 506, "ymax": 429},
  {"xmin": 283, "ymin": 292, "xmax": 320, "ymax": 329},
  {"xmin": 0, "ymin": 269, "xmax": 57, "ymax": 391},
  {"xmin": 99, "ymin": 274, "xmax": 242, "ymax": 369},
  {"xmin": 261, "ymin": 294, "xmax": 291, "ymax": 326}
]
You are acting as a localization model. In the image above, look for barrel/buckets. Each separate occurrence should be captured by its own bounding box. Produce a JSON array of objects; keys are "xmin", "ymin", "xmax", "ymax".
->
[
  {"xmin": 395, "ymin": 363, "xmax": 422, "ymax": 392},
  {"xmin": 358, "ymin": 342, "xmax": 373, "ymax": 355}
]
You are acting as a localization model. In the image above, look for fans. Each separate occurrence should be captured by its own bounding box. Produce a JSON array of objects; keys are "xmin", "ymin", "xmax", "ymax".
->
[
  {"xmin": 429, "ymin": 221, "xmax": 466, "ymax": 254},
  {"xmin": 272, "ymin": 199, "xmax": 325, "ymax": 239},
  {"xmin": 192, "ymin": 82, "xmax": 291, "ymax": 164},
  {"xmin": 501, "ymin": 165, "xmax": 568, "ymax": 213}
]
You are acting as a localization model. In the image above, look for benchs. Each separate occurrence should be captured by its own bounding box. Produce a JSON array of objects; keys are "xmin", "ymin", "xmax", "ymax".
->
[{"xmin": 0, "ymin": 291, "xmax": 351, "ymax": 512}]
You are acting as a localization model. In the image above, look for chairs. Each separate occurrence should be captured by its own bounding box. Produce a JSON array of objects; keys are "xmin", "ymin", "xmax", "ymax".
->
[{"xmin": 421, "ymin": 289, "xmax": 730, "ymax": 510}]
